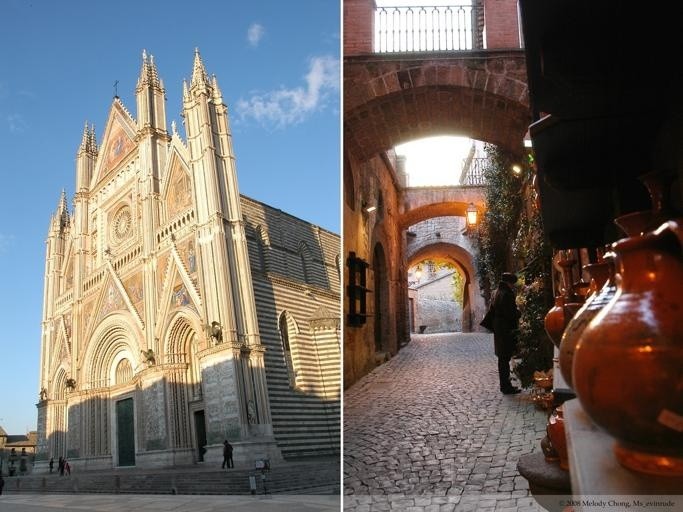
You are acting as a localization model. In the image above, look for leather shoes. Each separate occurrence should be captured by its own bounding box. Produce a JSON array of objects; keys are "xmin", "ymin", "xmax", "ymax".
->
[
  {"xmin": 503, "ymin": 389, "xmax": 521, "ymax": 395},
  {"xmin": 501, "ymin": 386, "xmax": 517, "ymax": 392}
]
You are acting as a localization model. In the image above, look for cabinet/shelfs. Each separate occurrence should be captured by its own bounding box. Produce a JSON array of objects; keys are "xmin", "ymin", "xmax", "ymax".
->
[{"xmin": 346, "ymin": 251, "xmax": 375, "ymax": 327}]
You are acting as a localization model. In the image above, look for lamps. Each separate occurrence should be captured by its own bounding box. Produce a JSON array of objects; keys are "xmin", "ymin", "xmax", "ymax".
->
[
  {"xmin": 362, "ymin": 201, "xmax": 376, "ymax": 212},
  {"xmin": 249, "ymin": 476, "xmax": 257, "ymax": 495},
  {"xmin": 464, "ymin": 202, "xmax": 478, "ymax": 230},
  {"xmin": 523, "ymin": 114, "xmax": 552, "ymax": 148},
  {"xmin": 255, "ymin": 459, "xmax": 272, "ymax": 495}
]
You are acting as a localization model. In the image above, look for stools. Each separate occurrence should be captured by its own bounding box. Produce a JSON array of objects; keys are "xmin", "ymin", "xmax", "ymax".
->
[{"xmin": 517, "ymin": 452, "xmax": 572, "ymax": 512}]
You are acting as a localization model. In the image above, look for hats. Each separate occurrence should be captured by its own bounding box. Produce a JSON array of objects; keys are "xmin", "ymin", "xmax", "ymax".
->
[{"xmin": 502, "ymin": 272, "xmax": 517, "ymax": 283}]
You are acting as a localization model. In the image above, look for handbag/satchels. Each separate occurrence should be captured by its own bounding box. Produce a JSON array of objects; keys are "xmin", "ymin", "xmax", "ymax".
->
[{"xmin": 479, "ymin": 306, "xmax": 496, "ymax": 331}]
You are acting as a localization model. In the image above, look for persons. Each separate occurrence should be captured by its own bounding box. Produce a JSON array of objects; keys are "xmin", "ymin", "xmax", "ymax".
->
[
  {"xmin": 220, "ymin": 441, "xmax": 230, "ymax": 468},
  {"xmin": 57, "ymin": 456, "xmax": 69, "ymax": 476},
  {"xmin": 488, "ymin": 272, "xmax": 524, "ymax": 395},
  {"xmin": 224, "ymin": 439, "xmax": 234, "ymax": 468},
  {"xmin": 0, "ymin": 475, "xmax": 4, "ymax": 495},
  {"xmin": 49, "ymin": 457, "xmax": 53, "ymax": 473}
]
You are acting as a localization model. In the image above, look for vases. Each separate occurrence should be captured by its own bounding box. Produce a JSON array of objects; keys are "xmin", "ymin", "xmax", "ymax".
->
[{"xmin": 541, "ymin": 168, "xmax": 683, "ymax": 476}]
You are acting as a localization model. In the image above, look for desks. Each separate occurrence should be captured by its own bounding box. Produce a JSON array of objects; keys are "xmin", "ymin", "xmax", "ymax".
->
[{"xmin": 562, "ymin": 398, "xmax": 683, "ymax": 495}]
